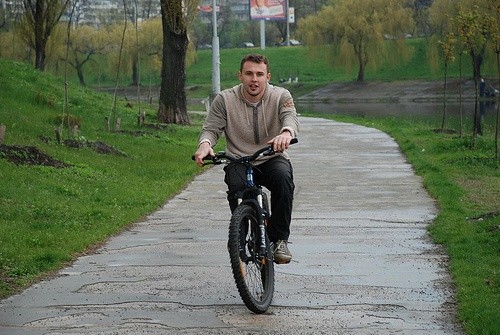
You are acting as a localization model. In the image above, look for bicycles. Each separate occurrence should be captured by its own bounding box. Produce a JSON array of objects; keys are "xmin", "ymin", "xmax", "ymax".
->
[{"xmin": 191, "ymin": 137, "xmax": 301, "ymax": 313}]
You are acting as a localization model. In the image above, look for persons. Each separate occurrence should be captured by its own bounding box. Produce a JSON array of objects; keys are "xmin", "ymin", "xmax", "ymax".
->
[{"xmin": 195, "ymin": 52, "xmax": 301, "ymax": 263}]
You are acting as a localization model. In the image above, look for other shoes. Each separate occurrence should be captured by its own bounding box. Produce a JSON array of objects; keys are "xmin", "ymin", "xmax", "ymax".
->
[
  {"xmin": 274, "ymin": 240, "xmax": 292, "ymax": 264},
  {"xmin": 237, "ymin": 239, "xmax": 246, "ymax": 254}
]
